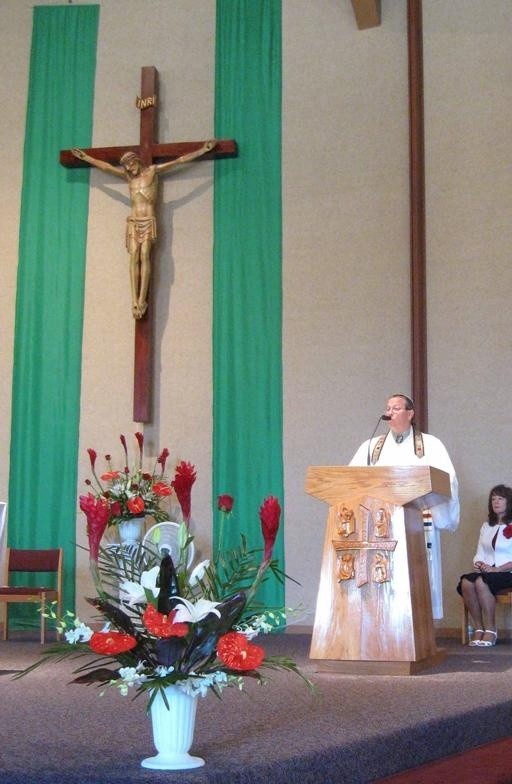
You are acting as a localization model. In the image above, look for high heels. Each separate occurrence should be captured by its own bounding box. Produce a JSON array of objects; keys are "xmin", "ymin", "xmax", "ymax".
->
[{"xmin": 468, "ymin": 629, "xmax": 497, "ymax": 647}]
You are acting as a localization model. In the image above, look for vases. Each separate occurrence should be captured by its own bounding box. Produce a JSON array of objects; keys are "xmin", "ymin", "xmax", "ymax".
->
[{"xmin": 139, "ymin": 688, "xmax": 208, "ymax": 770}]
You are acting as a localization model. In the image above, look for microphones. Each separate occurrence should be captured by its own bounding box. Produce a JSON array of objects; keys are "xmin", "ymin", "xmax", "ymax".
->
[{"xmin": 367, "ymin": 415, "xmax": 391, "ymax": 466}]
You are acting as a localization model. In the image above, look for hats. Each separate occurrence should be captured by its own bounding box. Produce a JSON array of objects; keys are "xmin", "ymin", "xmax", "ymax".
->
[{"xmin": 392, "ymin": 393, "xmax": 414, "ymax": 407}]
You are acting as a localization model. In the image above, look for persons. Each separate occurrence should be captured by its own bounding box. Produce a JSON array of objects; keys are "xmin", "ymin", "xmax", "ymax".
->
[
  {"xmin": 456, "ymin": 484, "xmax": 512, "ymax": 647},
  {"xmin": 347, "ymin": 393, "xmax": 461, "ymax": 620},
  {"xmin": 71, "ymin": 138, "xmax": 217, "ymax": 320}
]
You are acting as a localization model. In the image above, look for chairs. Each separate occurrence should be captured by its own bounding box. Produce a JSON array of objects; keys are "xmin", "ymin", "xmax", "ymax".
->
[
  {"xmin": 460, "ymin": 588, "xmax": 511, "ymax": 647},
  {"xmin": 0, "ymin": 547, "xmax": 63, "ymax": 645}
]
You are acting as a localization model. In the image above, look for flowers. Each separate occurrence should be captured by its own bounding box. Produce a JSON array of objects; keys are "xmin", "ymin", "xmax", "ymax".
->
[{"xmin": 29, "ymin": 432, "xmax": 316, "ymax": 700}]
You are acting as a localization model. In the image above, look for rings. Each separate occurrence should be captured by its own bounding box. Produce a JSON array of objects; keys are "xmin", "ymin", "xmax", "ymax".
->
[{"xmin": 484, "ymin": 567, "xmax": 486, "ymax": 569}]
